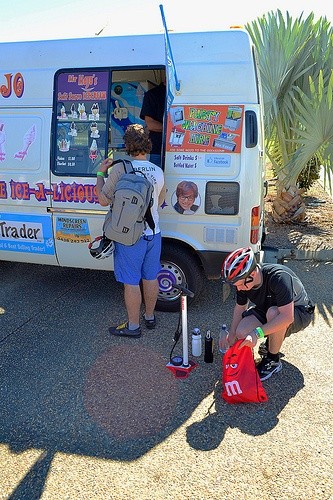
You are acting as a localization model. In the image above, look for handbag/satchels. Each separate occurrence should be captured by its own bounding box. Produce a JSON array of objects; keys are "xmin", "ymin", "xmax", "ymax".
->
[{"xmin": 221, "ymin": 340, "xmax": 269, "ymax": 403}]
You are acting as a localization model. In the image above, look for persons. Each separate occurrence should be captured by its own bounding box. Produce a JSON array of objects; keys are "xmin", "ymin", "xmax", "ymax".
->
[
  {"xmin": 139, "ymin": 70, "xmax": 166, "ymax": 172},
  {"xmin": 96, "ymin": 124, "xmax": 166, "ymax": 338},
  {"xmin": 174, "ymin": 181, "xmax": 199, "ymax": 215},
  {"xmin": 219, "ymin": 246, "xmax": 315, "ymax": 382}
]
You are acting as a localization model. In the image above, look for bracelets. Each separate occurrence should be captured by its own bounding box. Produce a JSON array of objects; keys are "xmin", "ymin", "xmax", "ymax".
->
[
  {"xmin": 256, "ymin": 327, "xmax": 264, "ymax": 337},
  {"xmin": 97, "ymin": 171, "xmax": 105, "ymax": 177}
]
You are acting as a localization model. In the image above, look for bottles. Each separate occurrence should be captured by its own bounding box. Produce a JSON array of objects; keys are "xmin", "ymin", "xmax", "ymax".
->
[
  {"xmin": 192, "ymin": 327, "xmax": 202, "ymax": 357},
  {"xmin": 219, "ymin": 324, "xmax": 230, "ymax": 353},
  {"xmin": 204, "ymin": 329, "xmax": 214, "ymax": 363}
]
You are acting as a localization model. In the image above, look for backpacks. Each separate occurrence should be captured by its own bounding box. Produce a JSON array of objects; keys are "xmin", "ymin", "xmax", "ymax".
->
[{"xmin": 101, "ymin": 159, "xmax": 156, "ymax": 246}]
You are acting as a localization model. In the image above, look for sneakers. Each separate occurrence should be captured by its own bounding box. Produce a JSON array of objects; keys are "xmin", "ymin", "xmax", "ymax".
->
[
  {"xmin": 108, "ymin": 320, "xmax": 142, "ymax": 338},
  {"xmin": 255, "ymin": 355, "xmax": 283, "ymax": 380},
  {"xmin": 142, "ymin": 311, "xmax": 156, "ymax": 328}
]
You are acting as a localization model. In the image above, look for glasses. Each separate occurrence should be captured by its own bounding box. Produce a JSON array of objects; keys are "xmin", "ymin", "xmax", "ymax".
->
[{"xmin": 181, "ymin": 196, "xmax": 193, "ymax": 201}]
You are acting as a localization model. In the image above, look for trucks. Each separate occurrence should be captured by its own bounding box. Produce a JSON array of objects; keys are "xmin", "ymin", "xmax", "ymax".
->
[{"xmin": 0, "ymin": 27, "xmax": 270, "ymax": 313}]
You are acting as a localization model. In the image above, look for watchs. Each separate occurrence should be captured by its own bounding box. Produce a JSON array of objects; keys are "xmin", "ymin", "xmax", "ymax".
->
[{"xmin": 254, "ymin": 329, "xmax": 260, "ymax": 338}]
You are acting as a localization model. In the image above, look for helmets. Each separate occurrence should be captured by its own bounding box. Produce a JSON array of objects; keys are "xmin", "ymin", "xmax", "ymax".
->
[
  {"xmin": 88, "ymin": 235, "xmax": 115, "ymax": 260},
  {"xmin": 220, "ymin": 246, "xmax": 258, "ymax": 289}
]
